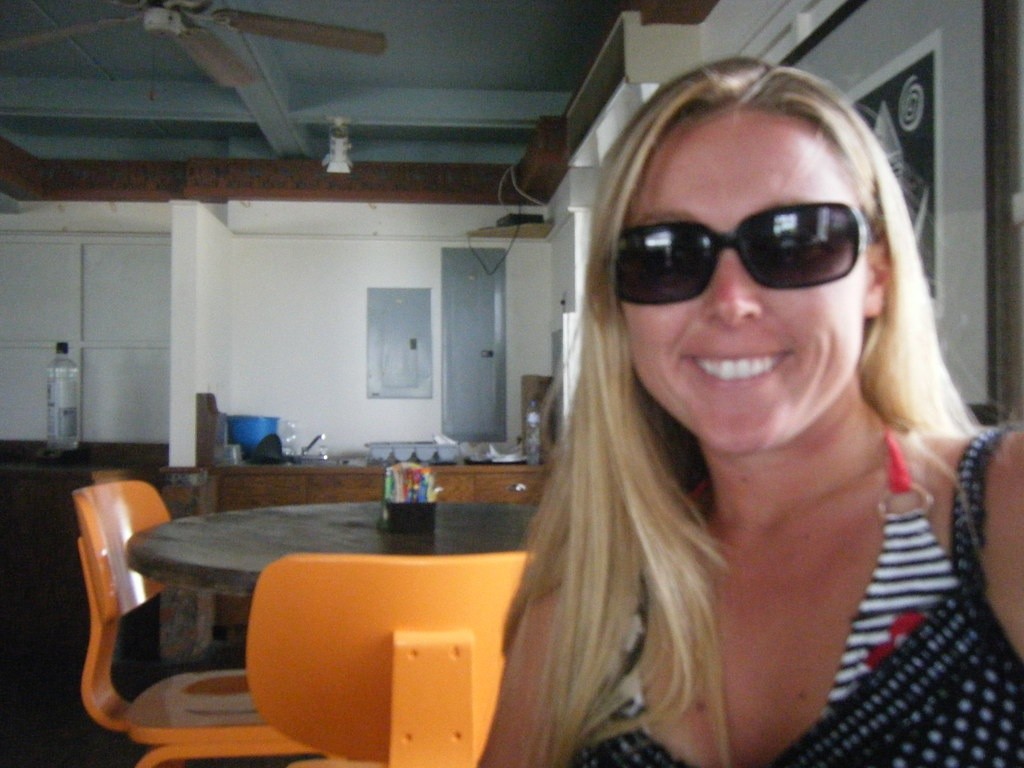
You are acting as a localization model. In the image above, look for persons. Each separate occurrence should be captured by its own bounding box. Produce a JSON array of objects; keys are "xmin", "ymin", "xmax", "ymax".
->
[{"xmin": 478, "ymin": 56, "xmax": 1024, "ymax": 768}]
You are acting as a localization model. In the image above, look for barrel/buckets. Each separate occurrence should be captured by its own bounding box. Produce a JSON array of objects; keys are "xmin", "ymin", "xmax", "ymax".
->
[{"xmin": 227, "ymin": 416, "xmax": 280, "ymax": 458}]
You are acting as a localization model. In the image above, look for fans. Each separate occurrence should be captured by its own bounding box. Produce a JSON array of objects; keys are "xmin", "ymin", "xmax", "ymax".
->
[{"xmin": 0, "ymin": 0, "xmax": 386, "ymax": 89}]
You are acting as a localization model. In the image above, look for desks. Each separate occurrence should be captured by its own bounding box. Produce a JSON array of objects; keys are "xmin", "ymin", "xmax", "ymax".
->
[{"xmin": 129, "ymin": 498, "xmax": 542, "ymax": 682}]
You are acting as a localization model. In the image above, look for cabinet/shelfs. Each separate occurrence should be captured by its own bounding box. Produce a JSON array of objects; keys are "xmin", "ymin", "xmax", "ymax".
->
[
  {"xmin": 220, "ymin": 474, "xmax": 542, "ymax": 624},
  {"xmin": 441, "ymin": 245, "xmax": 508, "ymax": 444},
  {"xmin": 366, "ymin": 288, "xmax": 433, "ymax": 398},
  {"xmin": 0, "ymin": 458, "xmax": 166, "ymax": 650}
]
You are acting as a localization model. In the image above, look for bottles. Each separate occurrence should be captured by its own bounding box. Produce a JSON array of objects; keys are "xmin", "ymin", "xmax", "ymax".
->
[
  {"xmin": 524, "ymin": 401, "xmax": 541, "ymax": 466},
  {"xmin": 44, "ymin": 342, "xmax": 81, "ymax": 450}
]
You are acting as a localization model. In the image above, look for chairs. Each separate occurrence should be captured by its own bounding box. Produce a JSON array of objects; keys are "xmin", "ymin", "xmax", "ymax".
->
[
  {"xmin": 244, "ymin": 552, "xmax": 533, "ymax": 768},
  {"xmin": 73, "ymin": 469, "xmax": 335, "ymax": 768}
]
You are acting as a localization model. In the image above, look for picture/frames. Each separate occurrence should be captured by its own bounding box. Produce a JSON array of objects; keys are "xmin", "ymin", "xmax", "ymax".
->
[{"xmin": 845, "ymin": 28, "xmax": 946, "ymax": 320}]
